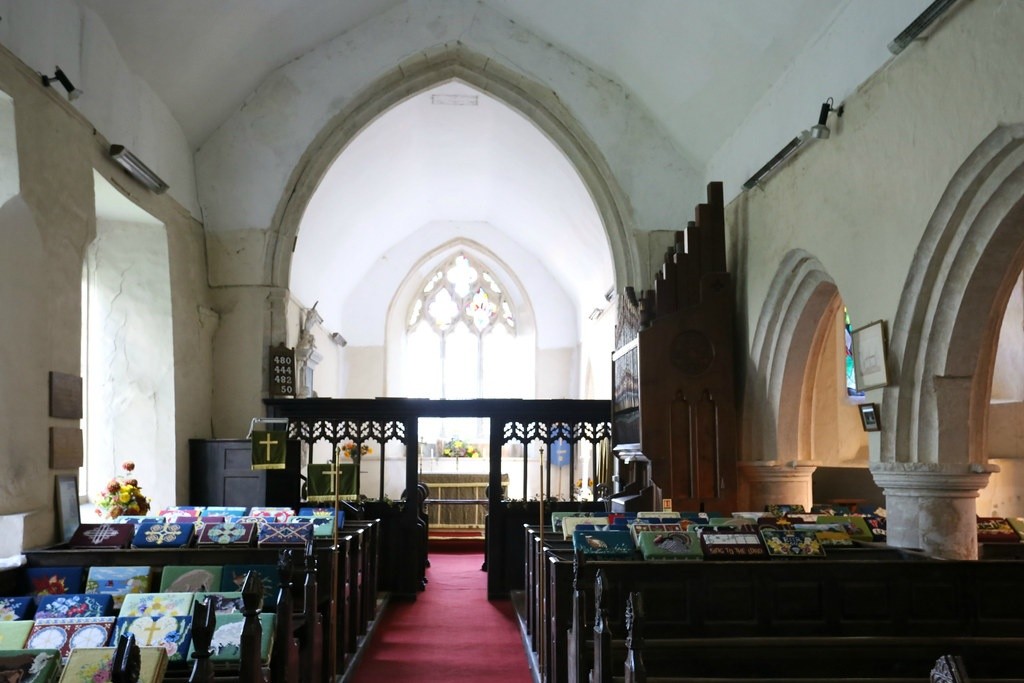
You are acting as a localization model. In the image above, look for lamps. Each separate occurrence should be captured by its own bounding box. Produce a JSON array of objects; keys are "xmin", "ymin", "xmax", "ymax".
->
[
  {"xmin": 858, "ymin": 402, "xmax": 880, "ymax": 431},
  {"xmin": 588, "ymin": 285, "xmax": 615, "ymax": 320},
  {"xmin": 333, "ymin": 333, "xmax": 347, "ymax": 347},
  {"xmin": 315, "ymin": 313, "xmax": 323, "ymax": 324},
  {"xmin": 740, "ymin": 129, "xmax": 810, "ymax": 191},
  {"xmin": 109, "ymin": 143, "xmax": 169, "ymax": 195},
  {"xmin": 887, "ymin": 0, "xmax": 957, "ymax": 55},
  {"xmin": 811, "ymin": 96, "xmax": 843, "ymax": 139},
  {"xmin": 42, "ymin": 65, "xmax": 84, "ymax": 100}
]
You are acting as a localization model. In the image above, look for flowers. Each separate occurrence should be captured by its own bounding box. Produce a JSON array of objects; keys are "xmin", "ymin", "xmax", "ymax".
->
[
  {"xmin": 343, "ymin": 442, "xmax": 372, "ymax": 458},
  {"xmin": 94, "ymin": 463, "xmax": 151, "ymax": 519},
  {"xmin": 443, "ymin": 439, "xmax": 480, "ymax": 459}
]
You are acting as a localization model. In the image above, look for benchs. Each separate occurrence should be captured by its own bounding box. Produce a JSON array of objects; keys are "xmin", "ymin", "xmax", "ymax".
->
[
  {"xmin": 0, "ymin": 515, "xmax": 385, "ymax": 683},
  {"xmin": 522, "ymin": 522, "xmax": 1023, "ymax": 682}
]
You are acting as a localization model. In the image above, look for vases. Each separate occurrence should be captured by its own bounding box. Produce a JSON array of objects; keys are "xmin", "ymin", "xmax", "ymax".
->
[{"xmin": 123, "ymin": 505, "xmax": 148, "ymax": 516}]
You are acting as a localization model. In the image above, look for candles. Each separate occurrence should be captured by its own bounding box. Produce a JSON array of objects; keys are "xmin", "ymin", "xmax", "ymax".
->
[{"xmin": 430, "ymin": 449, "xmax": 433, "ymax": 458}]
